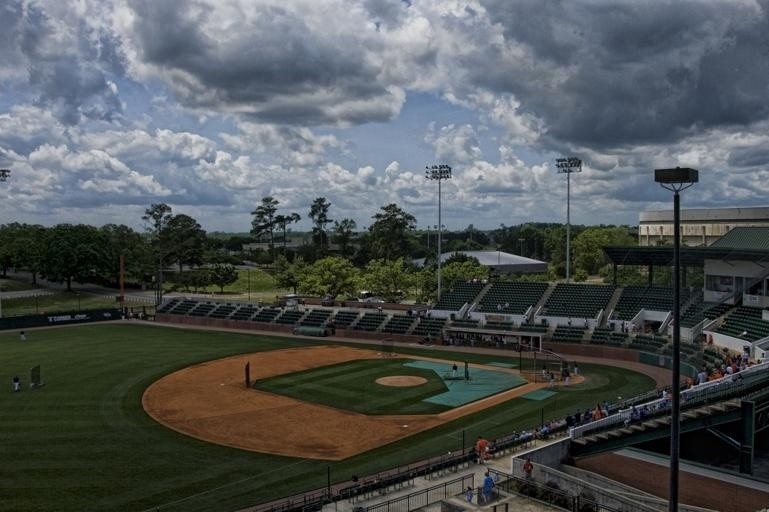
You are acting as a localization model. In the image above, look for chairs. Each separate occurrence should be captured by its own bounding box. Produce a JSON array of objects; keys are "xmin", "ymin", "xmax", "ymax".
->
[
  {"xmin": 270, "ymin": 370, "xmax": 769, "ymax": 512},
  {"xmin": 155, "ymin": 281, "xmax": 769, "ymax": 371}
]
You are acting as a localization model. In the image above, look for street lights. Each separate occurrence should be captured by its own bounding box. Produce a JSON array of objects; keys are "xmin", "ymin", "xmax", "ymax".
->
[
  {"xmin": 425, "ymin": 163, "xmax": 451, "ymax": 305},
  {"xmin": 554, "ymin": 156, "xmax": 583, "ymax": 283},
  {"xmin": 654, "ymin": 164, "xmax": 700, "ymax": 512}
]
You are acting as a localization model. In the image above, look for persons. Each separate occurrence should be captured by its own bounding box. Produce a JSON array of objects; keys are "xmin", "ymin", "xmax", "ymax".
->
[
  {"xmin": 568, "ymin": 316, "xmax": 572, "ymax": 326},
  {"xmin": 660, "ymin": 346, "xmax": 761, "ymax": 401},
  {"xmin": 584, "ymin": 319, "xmax": 589, "ymax": 328},
  {"xmin": 543, "ymin": 359, "xmax": 580, "ymax": 389},
  {"xmin": 19, "ymin": 330, "xmax": 25, "ymax": 340},
  {"xmin": 466, "ymin": 485, "xmax": 473, "ymax": 501},
  {"xmin": 621, "ymin": 319, "xmax": 654, "ymax": 334},
  {"xmin": 13, "ymin": 374, "xmax": 20, "ymax": 391},
  {"xmin": 510, "ymin": 399, "xmax": 610, "ymax": 451},
  {"xmin": 482, "ymin": 472, "xmax": 495, "ymax": 504},
  {"xmin": 452, "ymin": 363, "xmax": 457, "ymax": 376},
  {"xmin": 523, "ymin": 458, "xmax": 534, "ymax": 480},
  {"xmin": 476, "ymin": 434, "xmax": 489, "ymax": 464}
]
